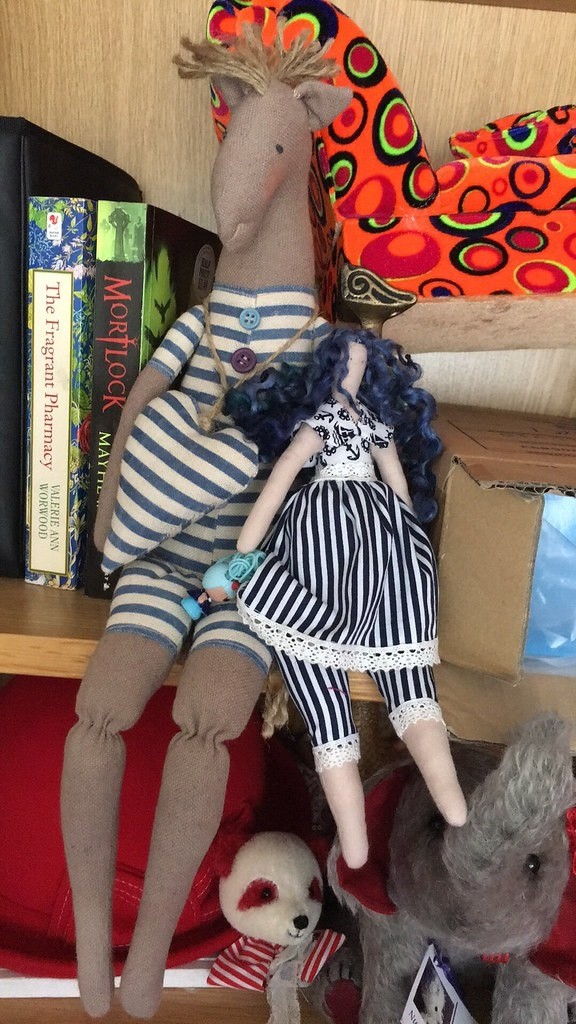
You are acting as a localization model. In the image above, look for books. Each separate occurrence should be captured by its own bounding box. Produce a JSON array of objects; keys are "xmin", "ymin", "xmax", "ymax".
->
[
  {"xmin": 21, "ymin": 192, "xmax": 101, "ymax": 592},
  {"xmin": 79, "ymin": 189, "xmax": 226, "ymax": 597},
  {"xmin": 2, "ymin": 112, "xmax": 144, "ymax": 579}
]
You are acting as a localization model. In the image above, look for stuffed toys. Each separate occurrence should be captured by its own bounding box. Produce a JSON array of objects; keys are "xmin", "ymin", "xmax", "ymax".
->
[
  {"xmin": 319, "ymin": 712, "xmax": 576, "ymax": 1023},
  {"xmin": 214, "ymin": 315, "xmax": 473, "ymax": 870},
  {"xmin": 44, "ymin": 3, "xmax": 358, "ymax": 1021},
  {"xmin": 208, "ymin": 820, "xmax": 347, "ymax": 994}
]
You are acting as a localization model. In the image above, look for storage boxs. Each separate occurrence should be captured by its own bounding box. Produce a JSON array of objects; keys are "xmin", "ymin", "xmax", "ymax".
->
[{"xmin": 430, "ymin": 408, "xmax": 576, "ymax": 757}]
